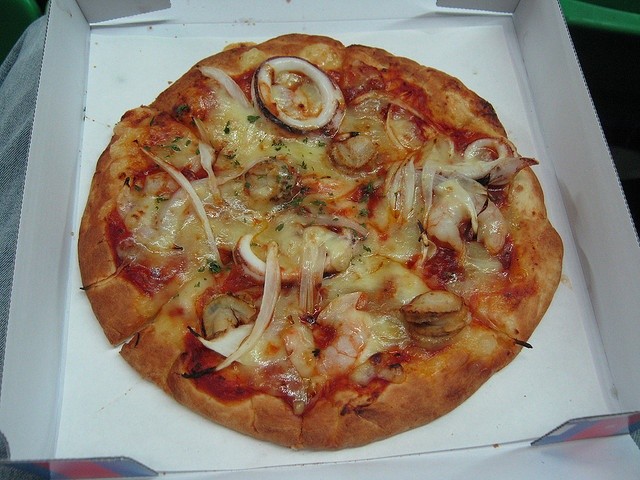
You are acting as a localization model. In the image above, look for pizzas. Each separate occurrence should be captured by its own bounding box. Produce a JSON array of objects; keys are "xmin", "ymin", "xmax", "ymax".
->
[{"xmin": 77, "ymin": 35, "xmax": 565, "ymax": 454}]
